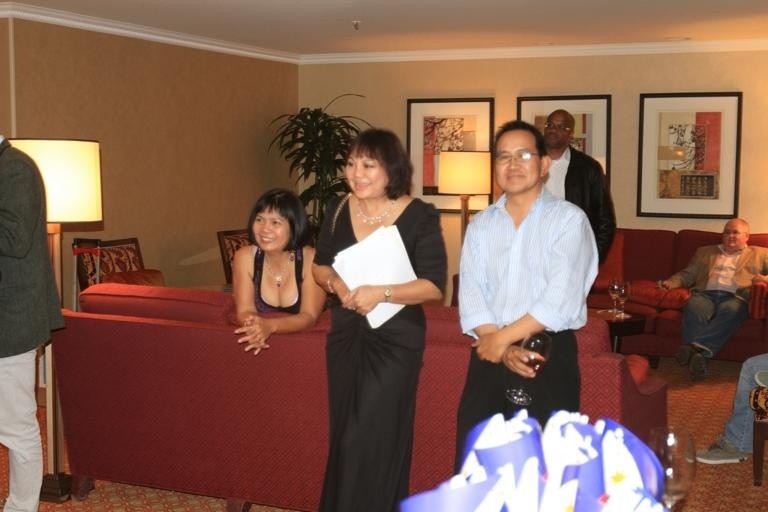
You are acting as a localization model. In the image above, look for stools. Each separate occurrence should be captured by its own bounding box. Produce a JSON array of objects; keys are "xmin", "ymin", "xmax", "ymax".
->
[{"xmin": 749, "ymin": 386, "xmax": 767, "ymax": 485}]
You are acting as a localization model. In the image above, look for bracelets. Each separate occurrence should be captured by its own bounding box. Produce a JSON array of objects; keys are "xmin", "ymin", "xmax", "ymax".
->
[{"xmin": 326, "ymin": 279, "xmax": 334, "ymax": 293}]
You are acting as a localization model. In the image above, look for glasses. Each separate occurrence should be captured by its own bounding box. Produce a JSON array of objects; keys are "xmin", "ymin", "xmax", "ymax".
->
[
  {"xmin": 493, "ymin": 149, "xmax": 539, "ymax": 164},
  {"xmin": 544, "ymin": 121, "xmax": 572, "ymax": 133}
]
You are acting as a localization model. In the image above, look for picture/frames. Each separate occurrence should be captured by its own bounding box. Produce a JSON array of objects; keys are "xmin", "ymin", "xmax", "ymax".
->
[
  {"xmin": 516, "ymin": 94, "xmax": 611, "ymax": 188},
  {"xmin": 405, "ymin": 97, "xmax": 495, "ymax": 214},
  {"xmin": 635, "ymin": 92, "xmax": 743, "ymax": 219}
]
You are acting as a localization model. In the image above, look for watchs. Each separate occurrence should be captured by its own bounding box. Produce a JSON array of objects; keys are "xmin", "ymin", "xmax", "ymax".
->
[{"xmin": 383, "ymin": 285, "xmax": 391, "ymax": 302}]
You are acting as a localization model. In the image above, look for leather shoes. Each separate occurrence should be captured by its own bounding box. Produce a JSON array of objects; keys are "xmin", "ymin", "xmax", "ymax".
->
[{"xmin": 677, "ymin": 344, "xmax": 708, "ymax": 382}]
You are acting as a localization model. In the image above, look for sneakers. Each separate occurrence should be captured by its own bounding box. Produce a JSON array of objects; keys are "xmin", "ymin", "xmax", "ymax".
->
[{"xmin": 695, "ymin": 436, "xmax": 747, "ymax": 465}]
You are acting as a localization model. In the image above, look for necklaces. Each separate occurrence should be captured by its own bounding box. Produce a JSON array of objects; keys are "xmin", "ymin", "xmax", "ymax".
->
[
  {"xmin": 354, "ymin": 198, "xmax": 397, "ymax": 224},
  {"xmin": 264, "ymin": 252, "xmax": 294, "ymax": 287}
]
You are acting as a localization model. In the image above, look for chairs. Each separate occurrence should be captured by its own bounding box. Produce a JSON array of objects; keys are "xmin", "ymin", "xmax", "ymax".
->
[
  {"xmin": 72, "ymin": 236, "xmax": 144, "ymax": 291},
  {"xmin": 217, "ymin": 229, "xmax": 252, "ymax": 286}
]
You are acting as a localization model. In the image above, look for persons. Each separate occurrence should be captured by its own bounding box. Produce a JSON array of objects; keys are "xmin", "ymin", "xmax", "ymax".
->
[
  {"xmin": 696, "ymin": 353, "xmax": 768, "ymax": 464},
  {"xmin": 453, "ymin": 121, "xmax": 599, "ymax": 478},
  {"xmin": 540, "ymin": 109, "xmax": 618, "ymax": 264},
  {"xmin": 659, "ymin": 218, "xmax": 768, "ymax": 372},
  {"xmin": 312, "ymin": 130, "xmax": 449, "ymax": 511},
  {"xmin": 232, "ymin": 188, "xmax": 327, "ymax": 356},
  {"xmin": 0, "ymin": 134, "xmax": 68, "ymax": 512}
]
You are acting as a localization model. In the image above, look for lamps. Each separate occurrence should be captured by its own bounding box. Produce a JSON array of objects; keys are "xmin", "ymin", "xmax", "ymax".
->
[
  {"xmin": 6, "ymin": 136, "xmax": 105, "ymax": 504},
  {"xmin": 437, "ymin": 149, "xmax": 493, "ymax": 274}
]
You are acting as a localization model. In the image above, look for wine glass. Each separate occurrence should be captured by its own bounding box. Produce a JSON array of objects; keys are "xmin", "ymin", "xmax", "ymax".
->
[
  {"xmin": 646, "ymin": 423, "xmax": 696, "ymax": 509},
  {"xmin": 607, "ymin": 277, "xmax": 632, "ymax": 319},
  {"xmin": 504, "ymin": 331, "xmax": 555, "ymax": 407}
]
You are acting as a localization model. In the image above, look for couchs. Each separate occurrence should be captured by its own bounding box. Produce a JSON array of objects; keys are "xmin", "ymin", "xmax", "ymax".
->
[
  {"xmin": 52, "ymin": 283, "xmax": 668, "ymax": 510},
  {"xmin": 587, "ymin": 227, "xmax": 768, "ymax": 361}
]
